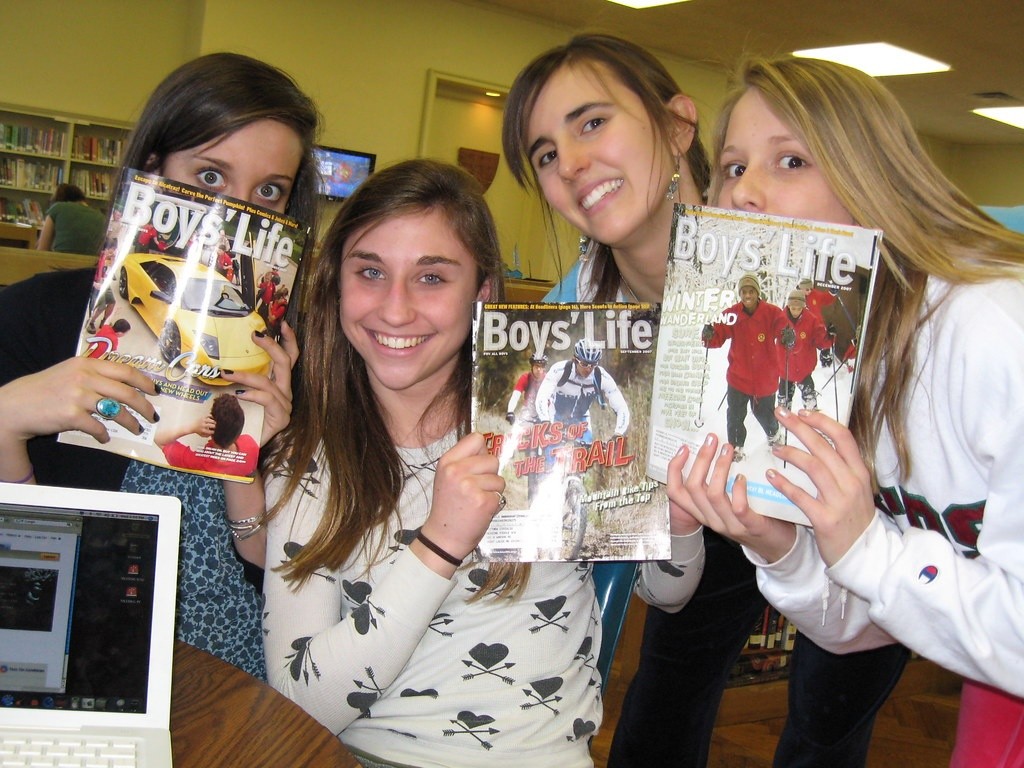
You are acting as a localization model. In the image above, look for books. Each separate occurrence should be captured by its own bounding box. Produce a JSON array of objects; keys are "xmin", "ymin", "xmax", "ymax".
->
[{"xmin": 1, "ymin": 124, "xmax": 129, "ymax": 226}]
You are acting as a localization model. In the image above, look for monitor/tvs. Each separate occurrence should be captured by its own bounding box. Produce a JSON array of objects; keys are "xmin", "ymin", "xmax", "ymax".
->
[{"xmin": 310, "ymin": 145, "xmax": 376, "ymax": 203}]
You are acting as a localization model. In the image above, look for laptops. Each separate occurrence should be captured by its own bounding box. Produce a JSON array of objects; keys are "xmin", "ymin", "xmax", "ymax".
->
[{"xmin": 0, "ymin": 481, "xmax": 181, "ymax": 767}]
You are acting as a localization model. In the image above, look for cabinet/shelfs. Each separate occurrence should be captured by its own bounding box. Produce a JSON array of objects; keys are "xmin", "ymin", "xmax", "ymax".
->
[{"xmin": 0, "ymin": 108, "xmax": 136, "ymax": 232}]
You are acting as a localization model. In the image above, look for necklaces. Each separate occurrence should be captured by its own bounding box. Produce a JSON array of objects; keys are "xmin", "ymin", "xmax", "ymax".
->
[{"xmin": 617, "ymin": 269, "xmax": 639, "ymax": 304}]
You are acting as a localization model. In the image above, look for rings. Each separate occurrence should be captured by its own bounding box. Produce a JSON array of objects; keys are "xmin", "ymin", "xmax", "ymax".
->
[
  {"xmin": 94, "ymin": 396, "xmax": 121, "ymax": 420},
  {"xmin": 494, "ymin": 490, "xmax": 507, "ymax": 513}
]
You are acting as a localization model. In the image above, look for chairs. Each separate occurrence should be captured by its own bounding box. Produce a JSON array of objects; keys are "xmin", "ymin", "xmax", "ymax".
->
[{"xmin": 0, "ymin": 222, "xmax": 38, "ymax": 250}]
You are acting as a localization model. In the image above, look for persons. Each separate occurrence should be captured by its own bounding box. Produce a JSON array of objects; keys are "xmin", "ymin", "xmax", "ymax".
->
[
  {"xmin": 0, "ymin": 53, "xmax": 321, "ymax": 686},
  {"xmin": 154, "ymin": 394, "xmax": 258, "ymax": 479},
  {"xmin": 501, "ymin": 29, "xmax": 910, "ymax": 768},
  {"xmin": 37, "ymin": 183, "xmax": 106, "ymax": 256},
  {"xmin": 670, "ymin": 57, "xmax": 1023, "ymax": 767},
  {"xmin": 701, "ymin": 274, "xmax": 851, "ymax": 461},
  {"xmin": 86, "ymin": 210, "xmax": 290, "ymax": 359},
  {"xmin": 505, "ymin": 338, "xmax": 630, "ymax": 506},
  {"xmin": 260, "ymin": 162, "xmax": 707, "ymax": 767}
]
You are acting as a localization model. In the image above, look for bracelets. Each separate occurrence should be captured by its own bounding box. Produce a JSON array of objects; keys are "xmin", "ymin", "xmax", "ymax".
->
[
  {"xmin": 225, "ymin": 515, "xmax": 263, "ymax": 540},
  {"xmin": 414, "ymin": 528, "xmax": 464, "ymax": 567},
  {"xmin": 1, "ymin": 464, "xmax": 35, "ymax": 483}
]
